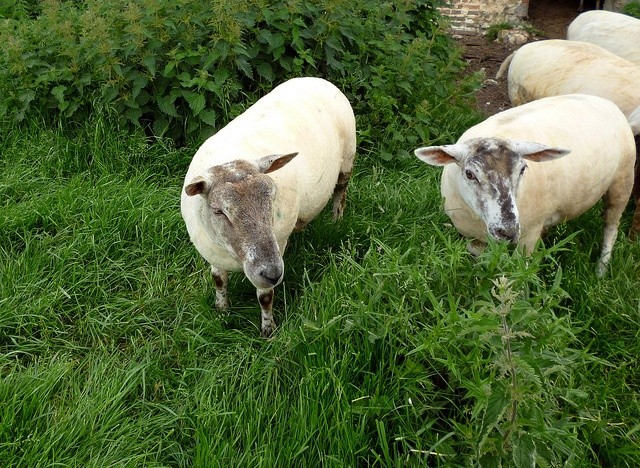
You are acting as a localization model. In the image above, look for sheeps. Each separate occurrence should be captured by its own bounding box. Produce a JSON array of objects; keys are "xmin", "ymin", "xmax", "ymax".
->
[
  {"xmin": 413, "ymin": 95, "xmax": 637, "ymax": 278},
  {"xmin": 567, "ymin": 10, "xmax": 640, "ymax": 64},
  {"xmin": 180, "ymin": 77, "xmax": 357, "ymax": 336},
  {"xmin": 496, "ymin": 39, "xmax": 640, "ymax": 136}
]
